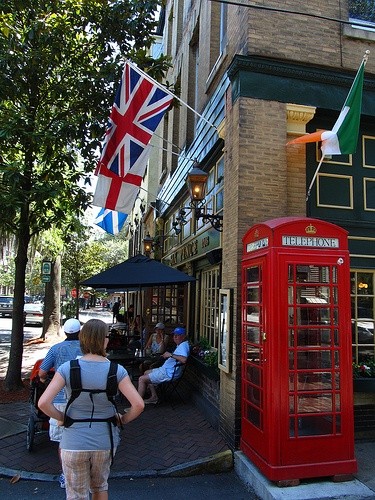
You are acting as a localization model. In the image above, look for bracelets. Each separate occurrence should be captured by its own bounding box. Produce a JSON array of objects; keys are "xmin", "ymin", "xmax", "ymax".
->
[{"xmin": 170, "ymin": 353, "xmax": 173, "ymax": 358}]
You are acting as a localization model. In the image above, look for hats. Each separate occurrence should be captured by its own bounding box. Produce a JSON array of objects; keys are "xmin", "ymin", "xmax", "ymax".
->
[
  {"xmin": 64, "ymin": 318, "xmax": 81, "ymax": 334},
  {"xmin": 155, "ymin": 323, "xmax": 165, "ymax": 330},
  {"xmin": 172, "ymin": 327, "xmax": 186, "ymax": 335}
]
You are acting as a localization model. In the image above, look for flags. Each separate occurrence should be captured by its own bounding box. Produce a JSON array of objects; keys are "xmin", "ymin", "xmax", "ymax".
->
[
  {"xmin": 100, "ymin": 60, "xmax": 173, "ymax": 179},
  {"xmin": 93, "ymin": 206, "xmax": 128, "ymax": 237},
  {"xmin": 285, "ymin": 57, "xmax": 366, "ymax": 156},
  {"xmin": 91, "ymin": 145, "xmax": 153, "ymax": 214}
]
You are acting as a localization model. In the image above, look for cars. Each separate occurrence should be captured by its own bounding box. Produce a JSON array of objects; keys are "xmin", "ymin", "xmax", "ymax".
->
[
  {"xmin": 24, "ymin": 295, "xmax": 45, "ymax": 303},
  {"xmin": 24, "ymin": 303, "xmax": 45, "ymax": 325},
  {"xmin": 0, "ymin": 295, "xmax": 14, "ymax": 317}
]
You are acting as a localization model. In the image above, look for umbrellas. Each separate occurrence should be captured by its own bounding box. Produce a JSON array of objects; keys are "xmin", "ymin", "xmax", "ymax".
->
[{"xmin": 78, "ymin": 254, "xmax": 200, "ymax": 375}]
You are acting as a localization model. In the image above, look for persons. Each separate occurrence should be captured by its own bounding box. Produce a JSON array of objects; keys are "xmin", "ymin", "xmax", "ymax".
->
[
  {"xmin": 39, "ymin": 319, "xmax": 98, "ymax": 493},
  {"xmin": 139, "ymin": 322, "xmax": 170, "ymax": 372},
  {"xmin": 129, "ymin": 315, "xmax": 141, "ymax": 353},
  {"xmin": 112, "ymin": 314, "xmax": 127, "ymax": 329},
  {"xmin": 112, "ymin": 300, "xmax": 121, "ymax": 324},
  {"xmin": 124, "ymin": 304, "xmax": 134, "ymax": 321},
  {"xmin": 38, "ymin": 319, "xmax": 145, "ymax": 500},
  {"xmin": 84, "ymin": 291, "xmax": 89, "ymax": 303},
  {"xmin": 124, "ymin": 328, "xmax": 190, "ymax": 413}
]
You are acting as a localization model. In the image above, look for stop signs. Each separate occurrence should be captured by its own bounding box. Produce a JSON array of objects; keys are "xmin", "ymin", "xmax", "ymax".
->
[
  {"xmin": 83, "ymin": 293, "xmax": 90, "ymax": 298},
  {"xmin": 71, "ymin": 288, "xmax": 80, "ymax": 297}
]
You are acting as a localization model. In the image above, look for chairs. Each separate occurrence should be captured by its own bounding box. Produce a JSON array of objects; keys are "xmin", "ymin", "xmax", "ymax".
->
[{"xmin": 154, "ymin": 356, "xmax": 193, "ymax": 410}]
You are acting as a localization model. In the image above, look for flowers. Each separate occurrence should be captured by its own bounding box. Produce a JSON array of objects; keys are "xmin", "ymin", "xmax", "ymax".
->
[
  {"xmin": 187, "ymin": 336, "xmax": 220, "ymax": 376},
  {"xmin": 353, "ymin": 356, "xmax": 375, "ymax": 383}
]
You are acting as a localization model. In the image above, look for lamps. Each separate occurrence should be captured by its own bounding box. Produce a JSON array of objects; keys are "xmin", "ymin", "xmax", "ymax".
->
[{"xmin": 141, "ymin": 157, "xmax": 224, "ymax": 253}]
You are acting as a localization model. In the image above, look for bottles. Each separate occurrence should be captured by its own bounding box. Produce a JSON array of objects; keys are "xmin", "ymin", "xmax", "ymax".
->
[{"xmin": 135, "ymin": 349, "xmax": 146, "ymax": 358}]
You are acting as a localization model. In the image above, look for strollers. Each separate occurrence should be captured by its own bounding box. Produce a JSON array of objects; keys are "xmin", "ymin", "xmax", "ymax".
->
[{"xmin": 26, "ymin": 358, "xmax": 55, "ymax": 452}]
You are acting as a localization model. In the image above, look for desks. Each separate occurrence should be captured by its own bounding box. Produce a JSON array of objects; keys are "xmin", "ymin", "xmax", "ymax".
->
[{"xmin": 106, "ymin": 323, "xmax": 158, "ymax": 402}]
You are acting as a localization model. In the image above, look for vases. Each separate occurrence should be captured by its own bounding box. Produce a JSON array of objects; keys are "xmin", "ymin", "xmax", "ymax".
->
[
  {"xmin": 353, "ymin": 377, "xmax": 375, "ymax": 392},
  {"xmin": 189, "ymin": 353, "xmax": 220, "ymax": 382}
]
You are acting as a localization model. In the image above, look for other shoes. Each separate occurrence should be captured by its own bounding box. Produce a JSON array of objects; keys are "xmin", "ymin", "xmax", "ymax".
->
[
  {"xmin": 143, "ymin": 398, "xmax": 157, "ymax": 404},
  {"xmin": 59, "ymin": 472, "xmax": 66, "ymax": 488},
  {"xmin": 124, "ymin": 408, "xmax": 131, "ymax": 412}
]
